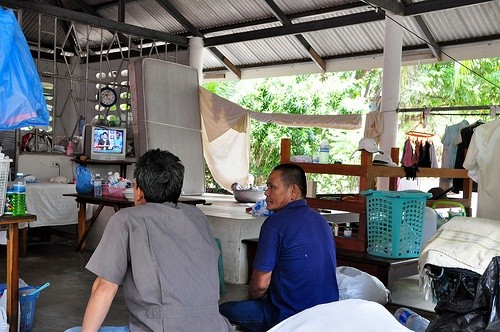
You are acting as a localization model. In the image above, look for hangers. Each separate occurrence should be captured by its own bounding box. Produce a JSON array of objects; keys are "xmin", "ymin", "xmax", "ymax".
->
[
  {"xmin": 462, "ymin": 115, "xmax": 469, "ymax": 121},
  {"xmin": 445, "ymin": 114, "xmax": 454, "ymax": 127},
  {"xmin": 477, "ymin": 113, "xmax": 485, "ymax": 122}
]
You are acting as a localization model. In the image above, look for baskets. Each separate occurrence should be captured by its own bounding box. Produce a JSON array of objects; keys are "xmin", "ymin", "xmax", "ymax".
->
[
  {"xmin": 0, "ymin": 280, "xmax": 53, "ymax": 332},
  {"xmin": 359, "ymin": 188, "xmax": 434, "ymax": 260},
  {"xmin": 0, "ymin": 158, "xmax": 14, "ymax": 216}
]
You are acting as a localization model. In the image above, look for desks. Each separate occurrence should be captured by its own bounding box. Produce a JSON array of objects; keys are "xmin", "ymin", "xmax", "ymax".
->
[
  {"xmin": 70, "ymin": 159, "xmax": 137, "ymax": 179},
  {"xmin": 0, "ymin": 211, "xmax": 37, "ymax": 332},
  {"xmin": 62, "ymin": 192, "xmax": 206, "ymax": 251},
  {"xmin": 241, "ymin": 238, "xmax": 429, "ymax": 303},
  {"xmin": 7, "ymin": 181, "xmax": 99, "ymax": 258}
]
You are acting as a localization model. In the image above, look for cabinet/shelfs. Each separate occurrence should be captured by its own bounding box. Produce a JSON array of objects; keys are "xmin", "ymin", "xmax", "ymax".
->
[{"xmin": 279, "ymin": 138, "xmax": 474, "ymax": 254}]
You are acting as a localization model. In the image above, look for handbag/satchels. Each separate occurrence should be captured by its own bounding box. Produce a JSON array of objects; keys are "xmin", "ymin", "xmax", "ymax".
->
[{"xmin": 75, "ymin": 164, "xmax": 94, "ymax": 195}]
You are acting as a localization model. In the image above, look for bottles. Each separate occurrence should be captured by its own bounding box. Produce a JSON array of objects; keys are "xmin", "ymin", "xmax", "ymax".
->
[
  {"xmin": 313, "ymin": 149, "xmax": 320, "ymax": 163},
  {"xmin": 332, "ymin": 222, "xmax": 339, "ymax": 237},
  {"xmin": 255, "ymin": 198, "xmax": 273, "ymax": 216},
  {"xmin": 108, "ymin": 172, "xmax": 120, "ymax": 189},
  {"xmin": 343, "ymin": 223, "xmax": 353, "ymax": 239},
  {"xmin": 94, "ymin": 174, "xmax": 102, "ymax": 198},
  {"xmin": 320, "ymin": 137, "xmax": 330, "ymax": 164},
  {"xmin": 13, "ymin": 173, "xmax": 26, "ymax": 216},
  {"xmin": 66, "ymin": 138, "xmax": 74, "ymax": 156},
  {"xmin": 393, "ymin": 307, "xmax": 430, "ymax": 332},
  {"xmin": 79, "ymin": 115, "xmax": 85, "ymax": 135}
]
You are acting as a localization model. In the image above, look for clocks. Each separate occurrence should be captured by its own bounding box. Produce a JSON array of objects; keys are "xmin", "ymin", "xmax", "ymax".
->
[{"xmin": 97, "ymin": 84, "xmax": 117, "ymax": 107}]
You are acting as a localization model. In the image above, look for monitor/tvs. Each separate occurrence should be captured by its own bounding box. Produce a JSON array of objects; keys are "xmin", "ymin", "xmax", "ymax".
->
[{"xmin": 84, "ymin": 125, "xmax": 127, "ymax": 160}]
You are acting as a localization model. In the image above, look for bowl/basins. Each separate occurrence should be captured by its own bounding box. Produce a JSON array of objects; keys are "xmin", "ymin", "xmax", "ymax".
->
[
  {"xmin": 107, "ymin": 186, "xmax": 126, "ymax": 199},
  {"xmin": 122, "ymin": 192, "xmax": 134, "ymax": 202},
  {"xmin": 232, "ymin": 189, "xmax": 267, "ymax": 202}
]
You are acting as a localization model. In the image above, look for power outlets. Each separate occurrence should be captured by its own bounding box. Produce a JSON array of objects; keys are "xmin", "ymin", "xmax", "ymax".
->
[{"xmin": 50, "ymin": 160, "xmax": 59, "ymax": 167}]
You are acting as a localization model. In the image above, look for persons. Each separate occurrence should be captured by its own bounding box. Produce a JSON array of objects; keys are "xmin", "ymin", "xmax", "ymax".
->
[
  {"xmin": 218, "ymin": 163, "xmax": 339, "ymax": 332},
  {"xmin": 64, "ymin": 148, "xmax": 237, "ymax": 332},
  {"xmin": 114, "ymin": 133, "xmax": 122, "ymax": 148},
  {"xmin": 98, "ymin": 132, "xmax": 110, "ymax": 150}
]
